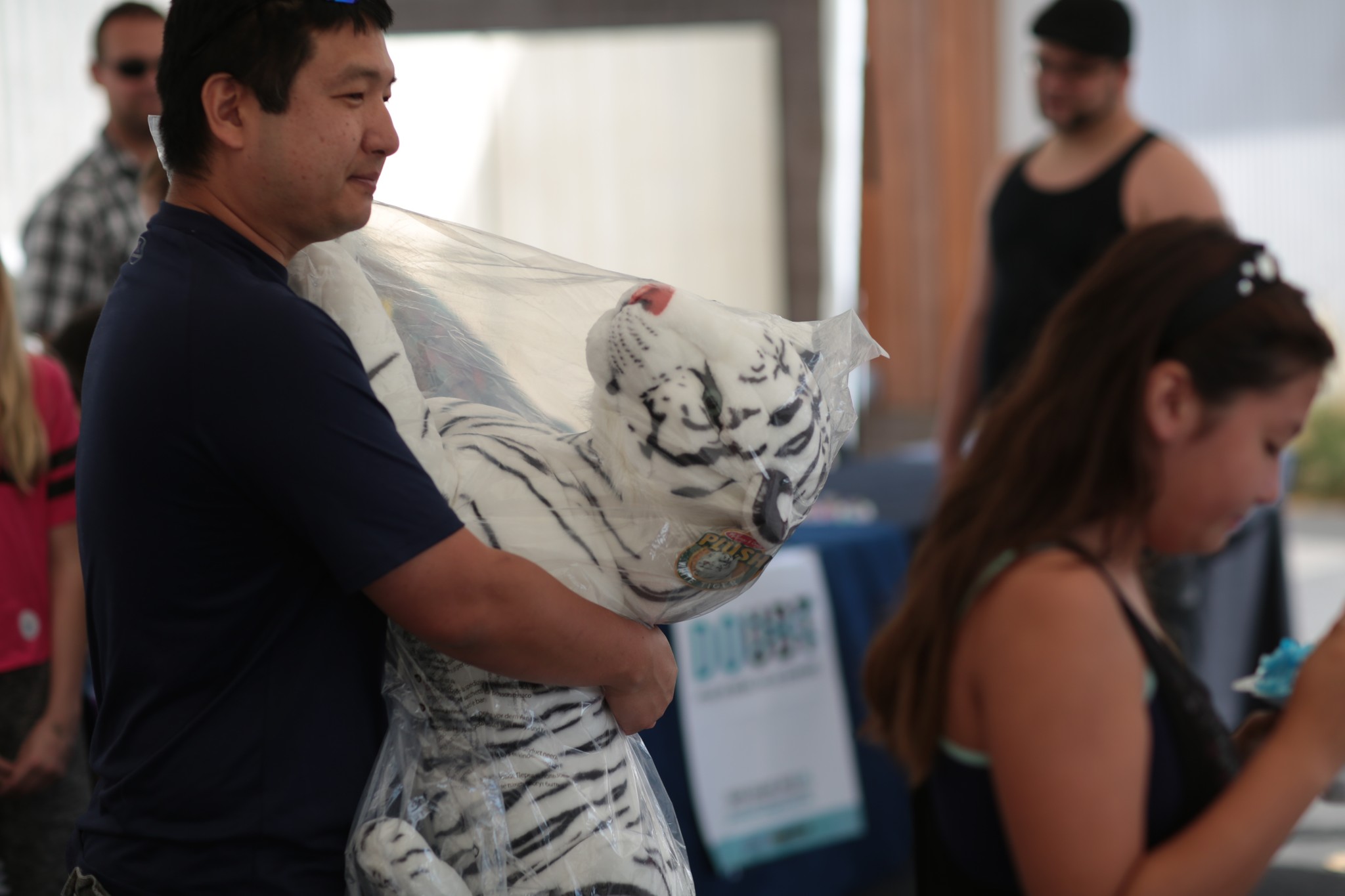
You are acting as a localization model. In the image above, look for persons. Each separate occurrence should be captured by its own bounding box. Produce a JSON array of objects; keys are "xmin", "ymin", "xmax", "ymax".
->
[
  {"xmin": 15, "ymin": 1, "xmax": 166, "ymax": 340},
  {"xmin": 937, "ymin": 0, "xmax": 1223, "ymax": 508},
  {"xmin": 46, "ymin": 151, "xmax": 174, "ymax": 805},
  {"xmin": 71, "ymin": 1, "xmax": 682, "ymax": 894},
  {"xmin": 0, "ymin": 252, "xmax": 85, "ymax": 896},
  {"xmin": 856, "ymin": 213, "xmax": 1344, "ymax": 896}
]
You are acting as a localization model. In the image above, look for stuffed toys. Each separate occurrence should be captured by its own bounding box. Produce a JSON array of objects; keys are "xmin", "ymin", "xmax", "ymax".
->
[{"xmin": 287, "ymin": 236, "xmax": 836, "ymax": 894}]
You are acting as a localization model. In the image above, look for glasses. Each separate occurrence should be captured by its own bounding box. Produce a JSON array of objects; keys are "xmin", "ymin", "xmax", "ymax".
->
[
  {"xmin": 104, "ymin": 56, "xmax": 160, "ymax": 80},
  {"xmin": 1175, "ymin": 241, "xmax": 1279, "ymax": 332}
]
